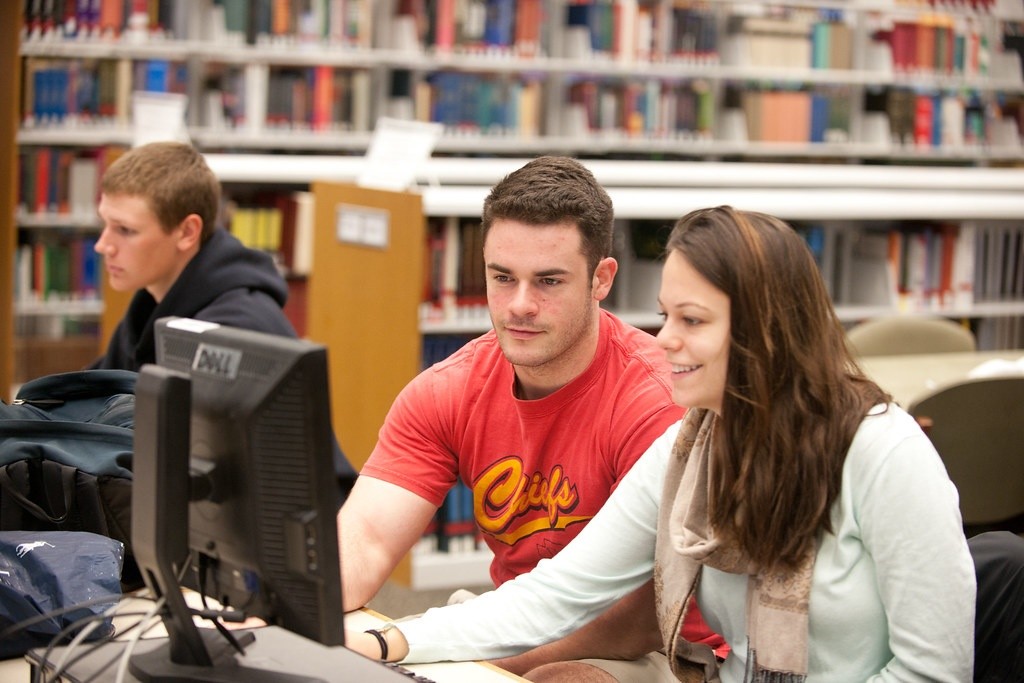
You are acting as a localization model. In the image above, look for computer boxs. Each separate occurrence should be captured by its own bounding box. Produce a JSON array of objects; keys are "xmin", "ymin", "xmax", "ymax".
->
[{"xmin": 23, "ymin": 624, "xmax": 417, "ymax": 683}]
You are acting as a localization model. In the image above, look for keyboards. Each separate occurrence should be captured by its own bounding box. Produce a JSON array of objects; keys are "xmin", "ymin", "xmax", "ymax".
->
[{"xmin": 386, "ymin": 661, "xmax": 435, "ymax": 683}]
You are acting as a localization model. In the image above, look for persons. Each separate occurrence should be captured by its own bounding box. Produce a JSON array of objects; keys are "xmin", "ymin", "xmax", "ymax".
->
[
  {"xmin": 339, "ymin": 155, "xmax": 687, "ymax": 681},
  {"xmin": 73, "ymin": 142, "xmax": 359, "ymax": 490},
  {"xmin": 344, "ymin": 205, "xmax": 977, "ymax": 683}
]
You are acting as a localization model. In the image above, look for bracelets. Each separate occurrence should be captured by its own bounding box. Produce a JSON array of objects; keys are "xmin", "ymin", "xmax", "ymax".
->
[{"xmin": 365, "ymin": 629, "xmax": 388, "ymax": 659}]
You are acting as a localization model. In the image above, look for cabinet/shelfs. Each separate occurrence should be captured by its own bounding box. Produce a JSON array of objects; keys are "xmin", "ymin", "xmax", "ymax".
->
[
  {"xmin": 97, "ymin": 144, "xmax": 1024, "ymax": 592},
  {"xmin": 0, "ymin": 0, "xmax": 1024, "ymax": 408}
]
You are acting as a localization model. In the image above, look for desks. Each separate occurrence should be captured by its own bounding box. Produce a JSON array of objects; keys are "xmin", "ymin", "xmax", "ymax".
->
[
  {"xmin": 1, "ymin": 587, "xmax": 540, "ymax": 683},
  {"xmin": 844, "ymin": 350, "xmax": 1024, "ymax": 413}
]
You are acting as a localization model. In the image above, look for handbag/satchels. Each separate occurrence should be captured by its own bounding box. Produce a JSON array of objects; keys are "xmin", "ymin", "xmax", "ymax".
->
[{"xmin": 0, "ymin": 368, "xmax": 147, "ymax": 596}]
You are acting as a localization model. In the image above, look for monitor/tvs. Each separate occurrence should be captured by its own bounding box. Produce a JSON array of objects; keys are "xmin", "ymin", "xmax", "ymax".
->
[{"xmin": 127, "ymin": 317, "xmax": 344, "ymax": 683}]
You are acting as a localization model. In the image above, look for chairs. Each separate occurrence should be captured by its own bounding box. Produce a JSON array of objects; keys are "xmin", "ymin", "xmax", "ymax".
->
[
  {"xmin": 844, "ymin": 316, "xmax": 974, "ymax": 355},
  {"xmin": 906, "ymin": 376, "xmax": 1023, "ymax": 525}
]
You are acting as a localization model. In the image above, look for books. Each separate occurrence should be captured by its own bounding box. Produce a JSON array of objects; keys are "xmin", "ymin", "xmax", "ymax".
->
[
  {"xmin": 376, "ymin": 0, "xmax": 723, "ymax": 62},
  {"xmin": 130, "ymin": 61, "xmax": 252, "ymax": 129},
  {"xmin": 980, "ymin": 225, "xmax": 1023, "ymax": 304},
  {"xmin": 860, "ymin": 9, "xmax": 1024, "ymax": 81},
  {"xmin": 787, "ymin": 221, "xmax": 975, "ymax": 309},
  {"xmin": 15, "ymin": 228, "xmax": 102, "ymax": 303},
  {"xmin": 408, "ymin": 479, "xmax": 490, "ymax": 554},
  {"xmin": 981, "ymin": 320, "xmax": 1024, "ymax": 354},
  {"xmin": 424, "ymin": 217, "xmax": 486, "ymax": 316},
  {"xmin": 865, "ymin": 89, "xmax": 1024, "ymax": 153},
  {"xmin": 265, "ymin": 65, "xmax": 379, "ymax": 133},
  {"xmin": 220, "ymin": 188, "xmax": 316, "ymax": 274},
  {"xmin": 723, "ymin": 81, "xmax": 852, "ymax": 143},
  {"xmin": 727, "ymin": 0, "xmax": 853, "ymax": 70},
  {"xmin": 19, "ymin": 57, "xmax": 117, "ymax": 135},
  {"xmin": 19, "ymin": 1, "xmax": 375, "ymax": 48},
  {"xmin": 16, "ymin": 143, "xmax": 129, "ymax": 216},
  {"xmin": 429, "ymin": 68, "xmax": 716, "ymax": 147}
]
